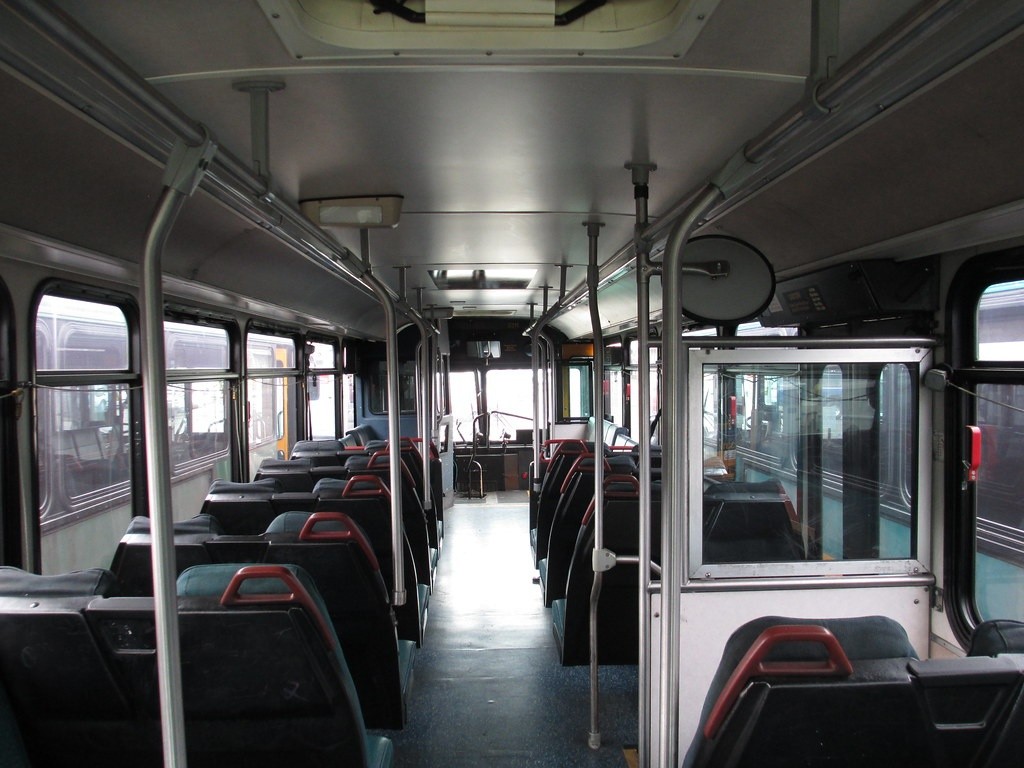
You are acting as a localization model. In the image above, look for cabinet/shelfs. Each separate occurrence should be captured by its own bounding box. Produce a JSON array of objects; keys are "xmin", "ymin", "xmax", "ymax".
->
[{"xmin": 456, "ymin": 454, "xmax": 519, "ymax": 492}]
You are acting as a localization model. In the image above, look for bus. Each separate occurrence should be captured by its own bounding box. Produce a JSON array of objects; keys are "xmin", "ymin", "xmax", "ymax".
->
[{"xmin": 0, "ymin": 0, "xmax": 1024, "ymax": 768}]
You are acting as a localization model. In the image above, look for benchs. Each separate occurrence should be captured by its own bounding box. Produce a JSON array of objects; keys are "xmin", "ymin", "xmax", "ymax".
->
[
  {"xmin": 681, "ymin": 617, "xmax": 1023, "ymax": 768},
  {"xmin": 529, "ymin": 414, "xmax": 806, "ymax": 668},
  {"xmin": 1, "ymin": 422, "xmax": 445, "ymax": 768}
]
